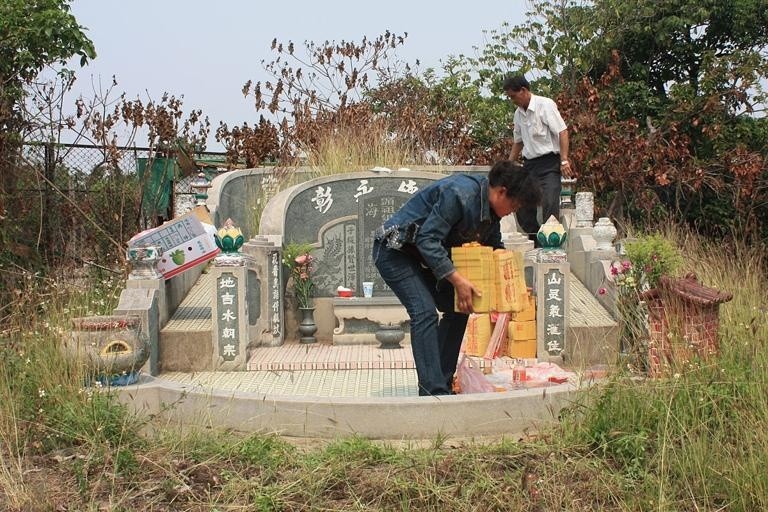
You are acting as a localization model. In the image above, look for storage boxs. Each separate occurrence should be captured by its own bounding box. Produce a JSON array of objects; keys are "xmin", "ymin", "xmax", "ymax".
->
[{"xmin": 128, "ymin": 204, "xmax": 221, "ymax": 280}]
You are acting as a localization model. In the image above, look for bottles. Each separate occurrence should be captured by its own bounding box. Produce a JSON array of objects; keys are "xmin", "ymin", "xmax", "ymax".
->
[{"xmin": 465, "ymin": 357, "xmax": 526, "ymax": 392}]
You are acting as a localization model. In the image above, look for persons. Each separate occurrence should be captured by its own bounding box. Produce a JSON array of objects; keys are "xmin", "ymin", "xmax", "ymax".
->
[
  {"xmin": 502, "ymin": 74, "xmax": 576, "ymax": 248},
  {"xmin": 370, "ymin": 157, "xmax": 543, "ymax": 397}
]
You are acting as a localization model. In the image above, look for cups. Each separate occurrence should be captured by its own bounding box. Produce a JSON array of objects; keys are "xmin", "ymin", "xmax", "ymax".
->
[
  {"xmin": 363, "ymin": 281, "xmax": 374, "ymax": 298},
  {"xmin": 338, "ymin": 291, "xmax": 353, "ymax": 298}
]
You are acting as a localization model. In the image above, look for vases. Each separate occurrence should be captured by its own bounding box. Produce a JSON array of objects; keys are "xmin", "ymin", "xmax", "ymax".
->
[{"xmin": 299, "ymin": 307, "xmax": 318, "ymax": 344}]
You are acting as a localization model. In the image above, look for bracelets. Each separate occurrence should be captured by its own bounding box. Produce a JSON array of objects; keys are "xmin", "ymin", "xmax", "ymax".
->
[{"xmin": 559, "ymin": 159, "xmax": 569, "ymax": 166}]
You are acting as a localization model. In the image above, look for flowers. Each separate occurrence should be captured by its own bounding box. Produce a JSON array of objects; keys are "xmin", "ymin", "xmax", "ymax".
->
[
  {"xmin": 282, "ymin": 236, "xmax": 321, "ymax": 308},
  {"xmin": 598, "ymin": 252, "xmax": 661, "ymax": 337}
]
